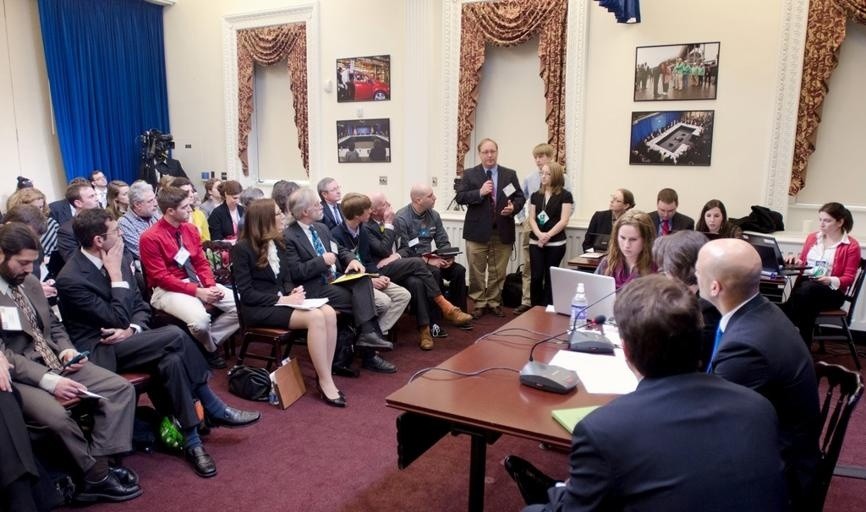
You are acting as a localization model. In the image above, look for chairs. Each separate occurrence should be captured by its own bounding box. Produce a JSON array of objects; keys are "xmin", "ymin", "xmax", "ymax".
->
[
  {"xmin": 813, "ymin": 360, "xmax": 865, "ymax": 512},
  {"xmin": 814, "ymin": 257, "xmax": 866, "ymax": 371},
  {"xmin": 202, "ymin": 240, "xmax": 236, "ymax": 358},
  {"xmin": 114, "ymin": 372, "xmax": 153, "ymax": 466},
  {"xmin": 23, "ymin": 396, "xmax": 82, "ymax": 479},
  {"xmin": 230, "ymin": 265, "xmax": 341, "ymax": 370}
]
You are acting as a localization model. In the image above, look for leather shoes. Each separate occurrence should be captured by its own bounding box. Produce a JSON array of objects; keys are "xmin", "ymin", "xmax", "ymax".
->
[
  {"xmin": 204, "ymin": 406, "xmax": 262, "ymax": 427},
  {"xmin": 76, "ymin": 462, "xmax": 144, "ymax": 502},
  {"xmin": 355, "ymin": 332, "xmax": 398, "ymax": 372},
  {"xmin": 185, "ymin": 446, "xmax": 217, "ymax": 476},
  {"xmin": 504, "ymin": 455, "xmax": 565, "ymax": 505}
]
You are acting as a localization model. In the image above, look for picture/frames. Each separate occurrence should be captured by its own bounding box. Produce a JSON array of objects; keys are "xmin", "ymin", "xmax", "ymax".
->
[
  {"xmin": 629, "ymin": 110, "xmax": 714, "ymax": 166},
  {"xmin": 336, "ymin": 118, "xmax": 391, "ymax": 163},
  {"xmin": 336, "ymin": 54, "xmax": 391, "ymax": 102},
  {"xmin": 634, "ymin": 41, "xmax": 720, "ymax": 102}
]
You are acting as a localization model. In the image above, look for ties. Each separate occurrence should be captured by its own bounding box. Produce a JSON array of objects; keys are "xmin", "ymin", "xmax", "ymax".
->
[
  {"xmin": 663, "ymin": 220, "xmax": 669, "ymax": 235},
  {"xmin": 706, "ymin": 316, "xmax": 724, "ymax": 375},
  {"xmin": 309, "ymin": 226, "xmax": 336, "ymax": 284},
  {"xmin": 176, "ymin": 231, "xmax": 196, "ymax": 282},
  {"xmin": 333, "ymin": 206, "xmax": 342, "ymax": 224},
  {"xmin": 488, "ymin": 177, "xmax": 496, "ymax": 226},
  {"xmin": 9, "ymin": 284, "xmax": 64, "ymax": 370},
  {"xmin": 379, "ymin": 221, "xmax": 385, "ymax": 234}
]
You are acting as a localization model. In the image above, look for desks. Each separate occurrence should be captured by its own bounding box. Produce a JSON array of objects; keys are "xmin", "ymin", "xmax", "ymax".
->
[
  {"xmin": 384, "ymin": 305, "xmax": 637, "ymax": 512},
  {"xmin": 567, "ymin": 251, "xmax": 787, "ymax": 302}
]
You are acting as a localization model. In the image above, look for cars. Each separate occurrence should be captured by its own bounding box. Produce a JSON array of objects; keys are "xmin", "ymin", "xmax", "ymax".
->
[
  {"xmin": 338, "ymin": 134, "xmax": 389, "ymax": 160},
  {"xmin": 338, "ymin": 67, "xmax": 390, "ymax": 101}
]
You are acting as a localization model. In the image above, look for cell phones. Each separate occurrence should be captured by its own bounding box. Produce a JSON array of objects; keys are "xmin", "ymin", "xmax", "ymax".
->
[{"xmin": 63, "ymin": 350, "xmax": 92, "ymax": 369}]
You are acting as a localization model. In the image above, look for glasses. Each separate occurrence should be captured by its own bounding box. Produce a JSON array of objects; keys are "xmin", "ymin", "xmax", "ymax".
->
[
  {"xmin": 275, "ymin": 209, "xmax": 282, "ymax": 217},
  {"xmin": 608, "ymin": 195, "xmax": 623, "ymax": 203},
  {"xmin": 481, "ymin": 150, "xmax": 497, "ymax": 154},
  {"xmin": 99, "ymin": 225, "xmax": 120, "ymax": 236}
]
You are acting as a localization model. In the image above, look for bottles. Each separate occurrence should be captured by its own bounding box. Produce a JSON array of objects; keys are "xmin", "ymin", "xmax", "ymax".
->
[
  {"xmin": 269, "ymin": 382, "xmax": 279, "ymax": 406},
  {"xmin": 570, "ymin": 283, "xmax": 588, "ymax": 331}
]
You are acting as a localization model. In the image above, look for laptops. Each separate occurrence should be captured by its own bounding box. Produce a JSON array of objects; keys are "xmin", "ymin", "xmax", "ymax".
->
[
  {"xmin": 740, "ymin": 232, "xmax": 813, "ymax": 269},
  {"xmin": 547, "ymin": 265, "xmax": 617, "ymax": 322},
  {"xmin": 586, "ymin": 231, "xmax": 611, "ymax": 254}
]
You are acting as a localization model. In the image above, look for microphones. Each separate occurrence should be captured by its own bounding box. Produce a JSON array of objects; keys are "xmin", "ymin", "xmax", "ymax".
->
[
  {"xmin": 519, "ymin": 314, "xmax": 607, "ymax": 393},
  {"xmin": 566, "ymin": 289, "xmax": 619, "ymax": 355},
  {"xmin": 486, "ymin": 169, "xmax": 493, "ymax": 181}
]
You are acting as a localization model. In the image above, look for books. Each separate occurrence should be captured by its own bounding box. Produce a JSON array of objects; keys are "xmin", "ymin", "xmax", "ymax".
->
[{"xmin": 552, "ymin": 406, "xmax": 603, "ymax": 435}]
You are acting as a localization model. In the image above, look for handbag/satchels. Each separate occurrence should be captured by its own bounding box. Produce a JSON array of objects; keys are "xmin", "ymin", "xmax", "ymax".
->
[
  {"xmin": 227, "ymin": 366, "xmax": 269, "ymax": 401},
  {"xmin": 502, "ymin": 264, "xmax": 522, "ymax": 307}
]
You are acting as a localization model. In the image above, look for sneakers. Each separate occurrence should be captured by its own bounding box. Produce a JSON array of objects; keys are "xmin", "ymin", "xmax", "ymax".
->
[
  {"xmin": 471, "ymin": 305, "xmax": 529, "ymax": 318},
  {"xmin": 420, "ymin": 306, "xmax": 473, "ymax": 349}
]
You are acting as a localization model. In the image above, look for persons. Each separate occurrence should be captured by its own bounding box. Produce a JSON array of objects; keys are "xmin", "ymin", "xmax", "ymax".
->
[
  {"xmin": 369, "ymin": 139, "xmax": 386, "ymax": 161},
  {"xmin": 529, "ymin": 162, "xmax": 573, "ymax": 307},
  {"xmin": 512, "ymin": 143, "xmax": 576, "ymax": 315},
  {"xmin": 1, "ymin": 170, "xmax": 262, "ymax": 503},
  {"xmin": 526, "ymin": 275, "xmax": 788, "ymax": 512},
  {"xmin": 581, "ymin": 187, "xmax": 743, "ymax": 372},
  {"xmin": 454, "ymin": 138, "xmax": 527, "ymax": 319},
  {"xmin": 504, "ymin": 238, "xmax": 822, "ymax": 512},
  {"xmin": 631, "ymin": 113, "xmax": 712, "ymax": 164},
  {"xmin": 636, "ymin": 57, "xmax": 711, "ymax": 98},
  {"xmin": 784, "ymin": 203, "xmax": 861, "ymax": 351},
  {"xmin": 209, "ymin": 178, "xmax": 474, "ymax": 408},
  {"xmin": 345, "ymin": 143, "xmax": 359, "ymax": 163}
]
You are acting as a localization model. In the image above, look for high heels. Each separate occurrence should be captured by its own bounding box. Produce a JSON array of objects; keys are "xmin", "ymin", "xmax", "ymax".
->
[{"xmin": 315, "ymin": 382, "xmax": 347, "ymax": 407}]
